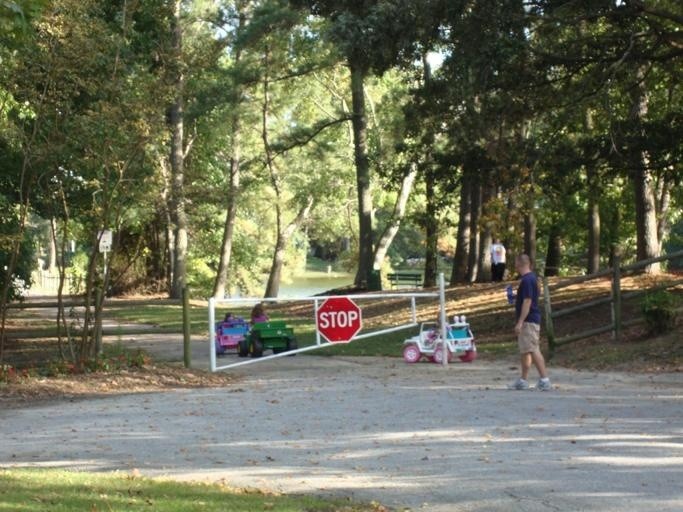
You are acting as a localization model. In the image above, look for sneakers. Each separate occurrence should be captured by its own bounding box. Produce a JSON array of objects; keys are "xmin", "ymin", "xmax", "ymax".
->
[
  {"xmin": 530, "ymin": 379, "xmax": 551, "ymax": 392},
  {"xmin": 508, "ymin": 378, "xmax": 529, "ymax": 390}
]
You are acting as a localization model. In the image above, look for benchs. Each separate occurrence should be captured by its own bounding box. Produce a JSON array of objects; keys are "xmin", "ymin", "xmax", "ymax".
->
[{"xmin": 387, "ymin": 273, "xmax": 423, "ymax": 291}]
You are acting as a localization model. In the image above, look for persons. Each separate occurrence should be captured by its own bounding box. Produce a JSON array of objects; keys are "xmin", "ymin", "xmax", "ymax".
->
[
  {"xmin": 491, "ymin": 238, "xmax": 507, "ymax": 281},
  {"xmin": 506, "ymin": 254, "xmax": 551, "ymax": 391},
  {"xmin": 252, "ymin": 304, "xmax": 268, "ymax": 323},
  {"xmin": 225, "ymin": 313, "xmax": 234, "ymax": 321}
]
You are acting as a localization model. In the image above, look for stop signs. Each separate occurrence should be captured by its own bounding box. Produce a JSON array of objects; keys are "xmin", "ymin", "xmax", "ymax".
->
[{"xmin": 316, "ymin": 297, "xmax": 362, "ymax": 344}]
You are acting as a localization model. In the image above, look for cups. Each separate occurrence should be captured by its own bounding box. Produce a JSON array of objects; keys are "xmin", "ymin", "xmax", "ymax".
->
[{"xmin": 507, "ymin": 287, "xmax": 513, "ymax": 304}]
